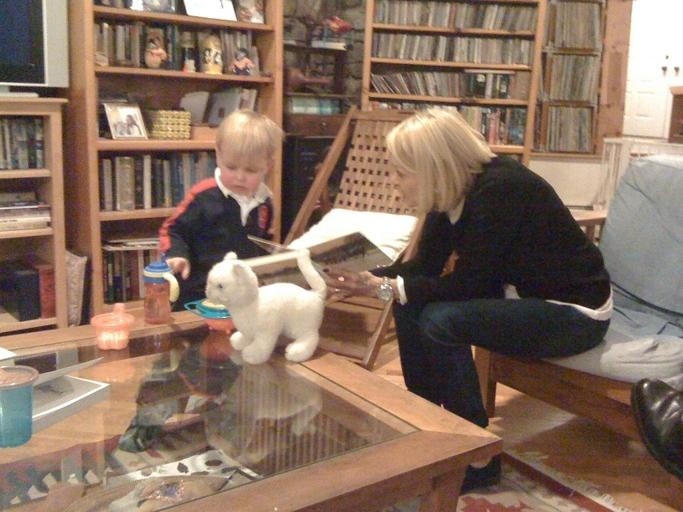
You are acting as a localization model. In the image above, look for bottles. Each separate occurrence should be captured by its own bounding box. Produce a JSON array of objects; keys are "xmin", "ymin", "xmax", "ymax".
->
[{"xmin": 139, "ymin": 259, "xmax": 181, "ymax": 324}]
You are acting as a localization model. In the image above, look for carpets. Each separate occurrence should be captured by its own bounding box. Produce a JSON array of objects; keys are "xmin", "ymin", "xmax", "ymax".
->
[{"xmin": 378, "ymin": 448, "xmax": 633, "ymax": 512}]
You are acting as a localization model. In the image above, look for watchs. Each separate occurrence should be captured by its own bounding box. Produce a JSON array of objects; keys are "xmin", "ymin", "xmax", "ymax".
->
[{"xmin": 377, "ymin": 276, "xmax": 394, "ymax": 303}]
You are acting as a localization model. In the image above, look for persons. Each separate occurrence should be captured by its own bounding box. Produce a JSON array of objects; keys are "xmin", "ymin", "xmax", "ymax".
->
[
  {"xmin": 116, "ymin": 108, "xmax": 284, "ymax": 455},
  {"xmin": 320, "ymin": 108, "xmax": 616, "ymax": 497}
]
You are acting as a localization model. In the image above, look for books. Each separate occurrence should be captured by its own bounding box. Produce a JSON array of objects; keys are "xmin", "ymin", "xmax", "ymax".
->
[
  {"xmin": 245, "ymin": 233, "xmax": 393, "ymax": 293},
  {"xmin": 0, "ymin": 116, "xmax": 51, "ymax": 231},
  {"xmin": 101, "ymin": 86, "xmax": 260, "ymax": 212},
  {"xmin": 371, "ymin": 0, "xmax": 599, "ymax": 152},
  {"xmin": 31, "ymin": 354, "xmax": 110, "ymax": 432},
  {"xmin": 94, "ymin": 0, "xmax": 264, "ymax": 77},
  {"xmin": 101, "ymin": 236, "xmax": 163, "ymax": 305}
]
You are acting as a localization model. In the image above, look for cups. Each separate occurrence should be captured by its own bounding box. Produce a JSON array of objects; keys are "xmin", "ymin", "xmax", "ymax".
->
[
  {"xmin": 90, "ymin": 301, "xmax": 135, "ymax": 352},
  {"xmin": 0, "ymin": 366, "xmax": 39, "ymax": 449}
]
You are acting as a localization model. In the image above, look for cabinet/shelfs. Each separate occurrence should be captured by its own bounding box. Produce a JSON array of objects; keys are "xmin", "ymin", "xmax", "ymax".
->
[{"xmin": 0, "ymin": 97, "xmax": 69, "ymax": 336}]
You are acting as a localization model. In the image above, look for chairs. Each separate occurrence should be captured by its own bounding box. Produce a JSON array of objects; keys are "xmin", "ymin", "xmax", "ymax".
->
[
  {"xmin": 258, "ymin": 104, "xmax": 433, "ymax": 371},
  {"xmin": 474, "ymin": 156, "xmax": 681, "ymax": 443}
]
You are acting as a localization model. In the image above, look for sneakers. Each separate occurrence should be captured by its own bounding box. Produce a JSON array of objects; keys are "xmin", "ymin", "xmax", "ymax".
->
[
  {"xmin": 119, "ymin": 412, "xmax": 161, "ymax": 451},
  {"xmin": 459, "ymin": 454, "xmax": 500, "ymax": 493}
]
